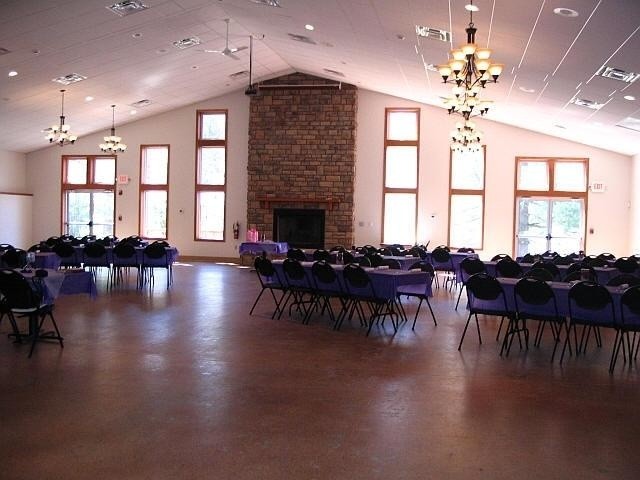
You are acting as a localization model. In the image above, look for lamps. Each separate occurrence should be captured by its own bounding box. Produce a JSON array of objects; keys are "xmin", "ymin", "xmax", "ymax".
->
[
  {"xmin": 436, "ymin": 0, "xmax": 504, "ymax": 153},
  {"xmin": 41, "ymin": 89, "xmax": 128, "ymax": 153}
]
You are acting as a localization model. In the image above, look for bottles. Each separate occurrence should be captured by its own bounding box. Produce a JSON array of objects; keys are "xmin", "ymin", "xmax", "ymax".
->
[
  {"xmin": 260, "ymin": 230, "xmax": 265, "ymax": 242},
  {"xmin": 246, "ymin": 227, "xmax": 258, "ymax": 243}
]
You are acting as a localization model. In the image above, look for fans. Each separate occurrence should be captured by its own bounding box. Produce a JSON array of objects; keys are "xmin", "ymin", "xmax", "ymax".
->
[{"xmin": 195, "ymin": 17, "xmax": 249, "ymax": 60}]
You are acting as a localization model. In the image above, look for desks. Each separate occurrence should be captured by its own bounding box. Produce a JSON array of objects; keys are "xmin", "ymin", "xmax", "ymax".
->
[{"xmin": 240, "ymin": 242, "xmax": 288, "ymax": 264}]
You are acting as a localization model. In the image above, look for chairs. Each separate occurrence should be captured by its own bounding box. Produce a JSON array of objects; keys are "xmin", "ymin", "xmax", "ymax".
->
[{"xmin": 0, "ymin": 233, "xmax": 169, "ymax": 358}]
